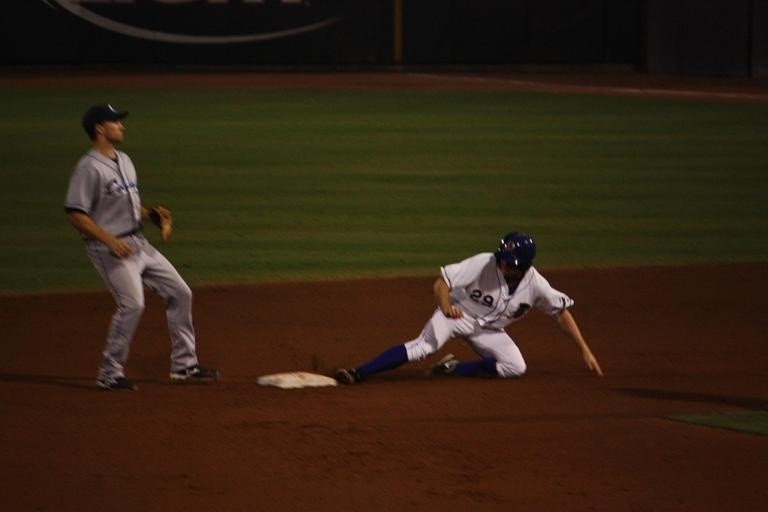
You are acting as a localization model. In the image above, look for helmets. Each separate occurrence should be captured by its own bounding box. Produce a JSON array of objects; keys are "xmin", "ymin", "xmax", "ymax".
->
[{"xmin": 495, "ymin": 232, "xmax": 536, "ymax": 268}]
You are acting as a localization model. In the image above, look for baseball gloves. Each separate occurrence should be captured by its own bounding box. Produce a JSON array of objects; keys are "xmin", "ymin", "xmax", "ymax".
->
[{"xmin": 146, "ymin": 202, "xmax": 174, "ymax": 242}]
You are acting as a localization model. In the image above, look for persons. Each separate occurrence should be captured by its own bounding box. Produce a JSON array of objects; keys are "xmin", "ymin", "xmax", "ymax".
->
[
  {"xmin": 333, "ymin": 229, "xmax": 606, "ymax": 390},
  {"xmin": 59, "ymin": 102, "xmax": 221, "ymax": 391}
]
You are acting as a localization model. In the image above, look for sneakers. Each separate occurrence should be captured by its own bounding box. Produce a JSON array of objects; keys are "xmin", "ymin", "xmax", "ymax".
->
[
  {"xmin": 426, "ymin": 353, "xmax": 458, "ymax": 379},
  {"xmin": 96, "ymin": 375, "xmax": 138, "ymax": 393},
  {"xmin": 170, "ymin": 365, "xmax": 220, "ymax": 382},
  {"xmin": 337, "ymin": 368, "xmax": 359, "ymax": 384}
]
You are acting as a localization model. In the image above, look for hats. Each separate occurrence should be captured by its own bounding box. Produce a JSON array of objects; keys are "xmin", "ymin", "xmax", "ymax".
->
[{"xmin": 81, "ymin": 103, "xmax": 129, "ymax": 133}]
